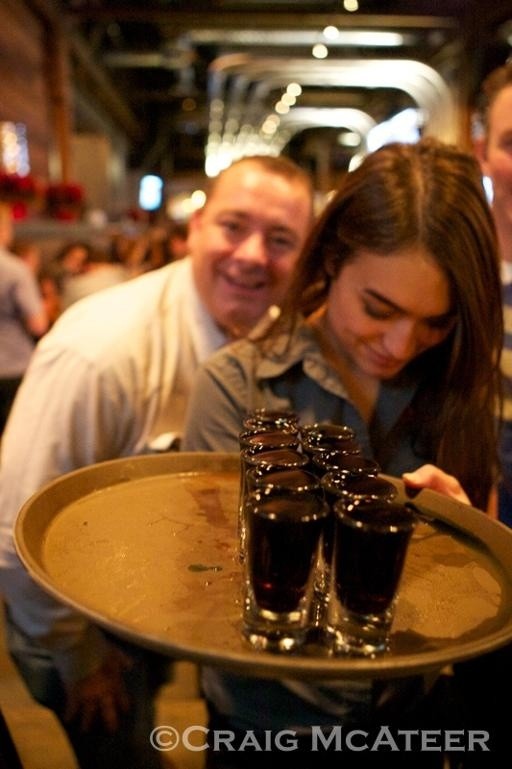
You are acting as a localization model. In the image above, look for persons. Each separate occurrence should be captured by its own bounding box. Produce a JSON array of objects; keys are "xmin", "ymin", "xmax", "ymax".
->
[
  {"xmin": 0, "ymin": 204, "xmax": 189, "ymax": 425},
  {"xmin": 193, "ymin": 140, "xmax": 503, "ymax": 769},
  {"xmin": 3, "ymin": 153, "xmax": 322, "ymax": 768},
  {"xmin": 469, "ymin": 59, "xmax": 511, "ymax": 529}
]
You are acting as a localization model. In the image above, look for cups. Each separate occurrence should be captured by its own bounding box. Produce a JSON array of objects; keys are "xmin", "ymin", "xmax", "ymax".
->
[{"xmin": 235, "ymin": 407, "xmax": 419, "ymax": 659}]
